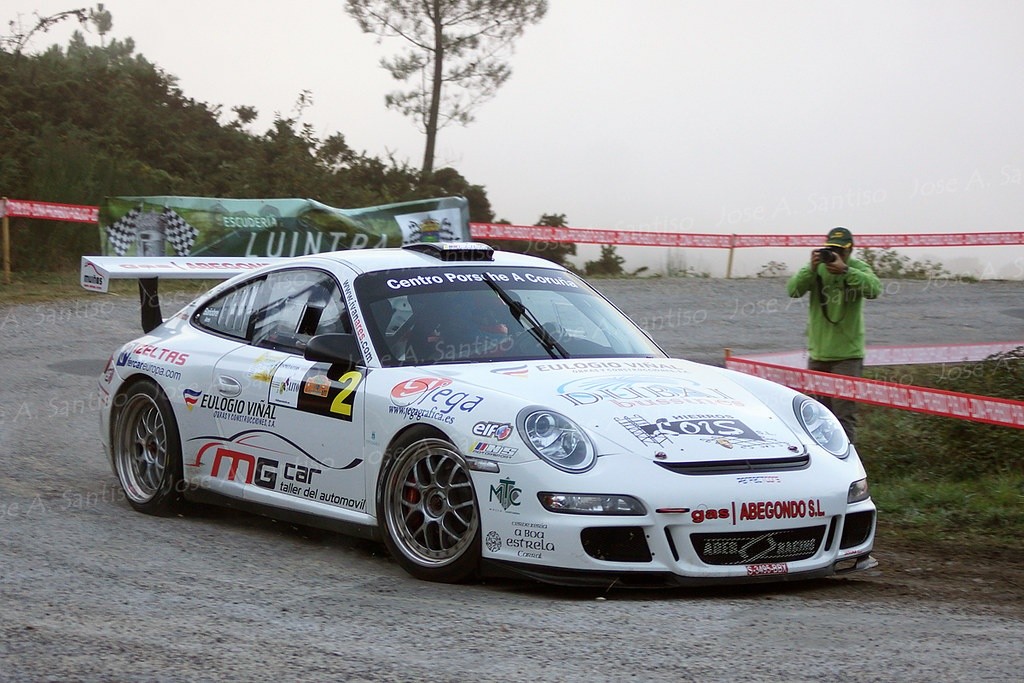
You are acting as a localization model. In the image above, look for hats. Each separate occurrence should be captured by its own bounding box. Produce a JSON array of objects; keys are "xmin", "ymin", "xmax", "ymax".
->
[{"xmin": 824, "ymin": 227, "xmax": 853, "ymax": 248}]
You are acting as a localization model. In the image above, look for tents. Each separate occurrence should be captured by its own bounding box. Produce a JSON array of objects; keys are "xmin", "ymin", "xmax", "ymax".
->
[{"xmin": 98, "ymin": 195, "xmax": 471, "ymax": 261}]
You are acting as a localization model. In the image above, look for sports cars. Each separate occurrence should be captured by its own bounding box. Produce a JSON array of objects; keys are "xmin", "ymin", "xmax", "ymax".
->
[{"xmin": 97, "ymin": 240, "xmax": 878, "ymax": 592}]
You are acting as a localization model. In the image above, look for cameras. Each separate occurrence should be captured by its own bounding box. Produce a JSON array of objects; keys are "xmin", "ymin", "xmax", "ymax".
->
[{"xmin": 816, "ymin": 248, "xmax": 837, "ymax": 263}]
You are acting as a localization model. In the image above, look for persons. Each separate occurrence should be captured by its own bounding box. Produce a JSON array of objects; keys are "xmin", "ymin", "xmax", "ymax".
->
[
  {"xmin": 787, "ymin": 227, "xmax": 884, "ymax": 446},
  {"xmin": 461, "ymin": 291, "xmax": 569, "ymax": 360}
]
